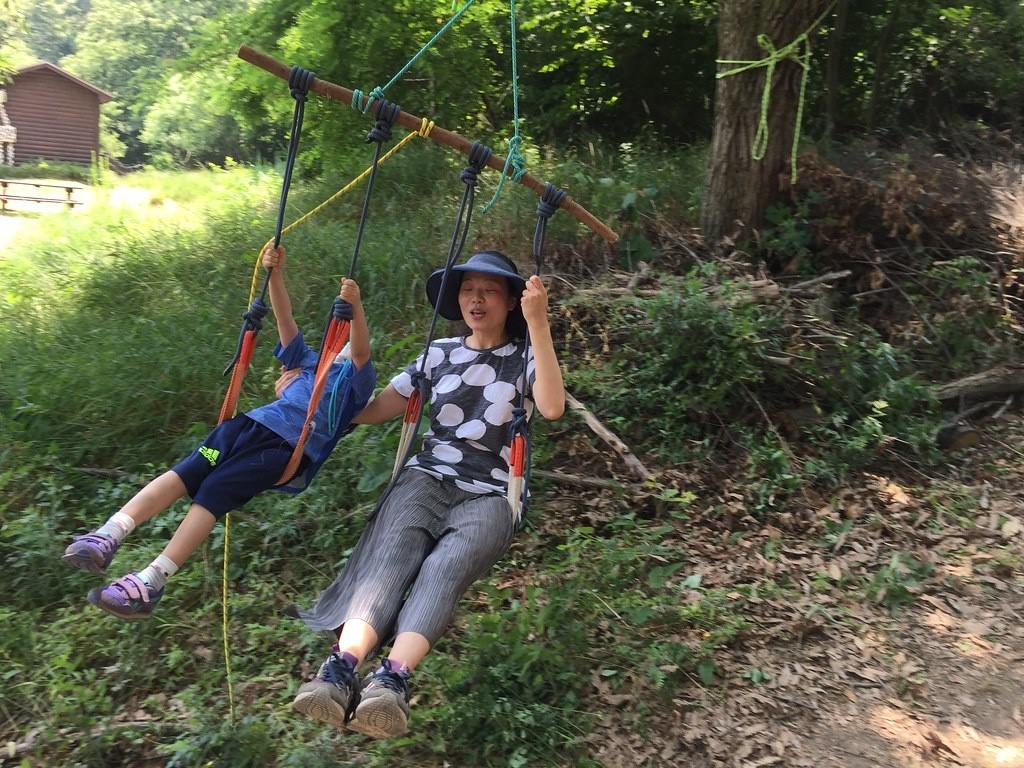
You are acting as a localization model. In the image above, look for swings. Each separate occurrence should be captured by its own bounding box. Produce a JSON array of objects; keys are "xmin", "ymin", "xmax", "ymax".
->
[
  {"xmin": 215, "ymin": 101, "xmax": 381, "ymax": 488},
  {"xmin": 389, "ymin": 184, "xmax": 549, "ymax": 535}
]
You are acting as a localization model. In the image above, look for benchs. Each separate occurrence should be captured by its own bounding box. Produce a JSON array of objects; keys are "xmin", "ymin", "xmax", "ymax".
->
[{"xmin": 0, "ymin": 194, "xmax": 82, "ymax": 208}]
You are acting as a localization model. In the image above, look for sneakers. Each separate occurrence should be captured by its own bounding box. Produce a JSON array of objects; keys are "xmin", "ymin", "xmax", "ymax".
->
[
  {"xmin": 87, "ymin": 572, "xmax": 164, "ymax": 619},
  {"xmin": 346, "ymin": 657, "xmax": 414, "ymax": 739},
  {"xmin": 294, "ymin": 644, "xmax": 361, "ymax": 727},
  {"xmin": 62, "ymin": 532, "xmax": 120, "ymax": 576}
]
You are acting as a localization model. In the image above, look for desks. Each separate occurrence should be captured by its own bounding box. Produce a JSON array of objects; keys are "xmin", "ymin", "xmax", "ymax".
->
[{"xmin": 0, "ymin": 179, "xmax": 83, "ymax": 209}]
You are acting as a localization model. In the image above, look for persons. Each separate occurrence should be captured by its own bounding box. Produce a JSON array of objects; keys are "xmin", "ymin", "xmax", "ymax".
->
[
  {"xmin": 61, "ymin": 243, "xmax": 375, "ymax": 618},
  {"xmin": 274, "ymin": 250, "xmax": 565, "ymax": 740}
]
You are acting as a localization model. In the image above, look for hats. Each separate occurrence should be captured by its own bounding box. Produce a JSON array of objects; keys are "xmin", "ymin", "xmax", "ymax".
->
[{"xmin": 426, "ymin": 251, "xmax": 530, "ymax": 339}]
